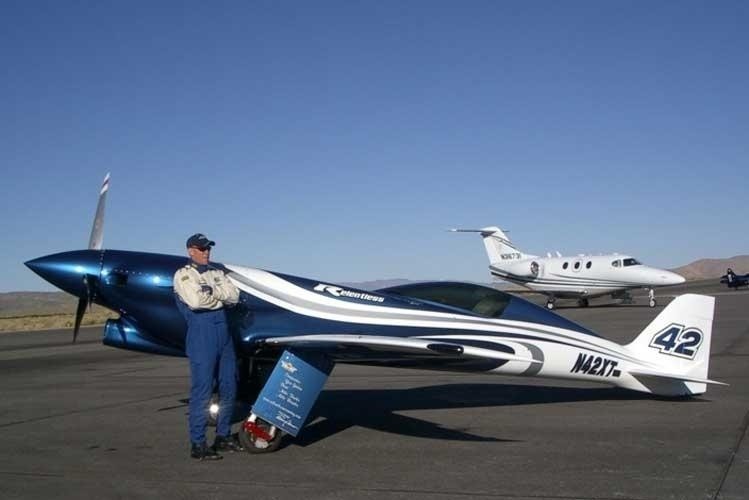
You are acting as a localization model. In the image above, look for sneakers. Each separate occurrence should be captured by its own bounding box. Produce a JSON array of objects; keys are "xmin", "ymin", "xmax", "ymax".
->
[
  {"xmin": 213, "ymin": 434, "xmax": 245, "ymax": 452},
  {"xmin": 190, "ymin": 443, "xmax": 223, "ymax": 461}
]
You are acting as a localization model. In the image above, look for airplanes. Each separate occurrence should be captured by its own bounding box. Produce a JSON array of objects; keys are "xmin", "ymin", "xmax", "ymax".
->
[{"xmin": 447, "ymin": 226, "xmax": 686, "ymax": 309}]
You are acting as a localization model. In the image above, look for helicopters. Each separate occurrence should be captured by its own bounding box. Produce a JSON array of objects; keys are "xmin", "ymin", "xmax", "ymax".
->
[{"xmin": 22, "ymin": 177, "xmax": 731, "ymax": 456}]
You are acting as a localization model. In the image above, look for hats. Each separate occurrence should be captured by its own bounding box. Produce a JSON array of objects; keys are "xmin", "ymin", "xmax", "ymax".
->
[{"xmin": 186, "ymin": 233, "xmax": 215, "ymax": 248}]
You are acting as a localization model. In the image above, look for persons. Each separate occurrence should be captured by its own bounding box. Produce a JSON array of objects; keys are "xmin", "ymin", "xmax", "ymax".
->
[
  {"xmin": 727, "ymin": 267, "xmax": 738, "ymax": 290},
  {"xmin": 172, "ymin": 233, "xmax": 244, "ymax": 460}
]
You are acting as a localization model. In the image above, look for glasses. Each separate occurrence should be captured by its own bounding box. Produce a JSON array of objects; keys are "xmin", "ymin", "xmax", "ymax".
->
[{"xmin": 191, "ymin": 246, "xmax": 211, "ymax": 251}]
